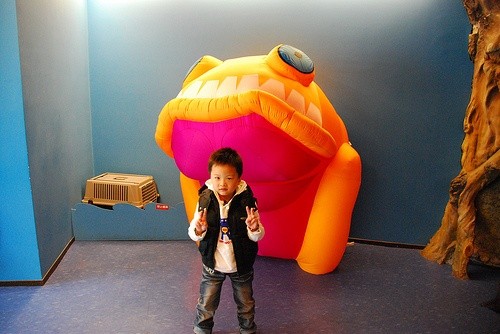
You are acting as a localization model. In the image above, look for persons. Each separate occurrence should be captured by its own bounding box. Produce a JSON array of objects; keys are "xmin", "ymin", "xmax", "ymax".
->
[{"xmin": 187, "ymin": 147, "xmax": 265, "ymax": 334}]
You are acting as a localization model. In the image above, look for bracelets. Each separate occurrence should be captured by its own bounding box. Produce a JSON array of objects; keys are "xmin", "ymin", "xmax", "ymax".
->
[{"xmin": 249, "ymin": 224, "xmax": 259, "ymax": 232}]
